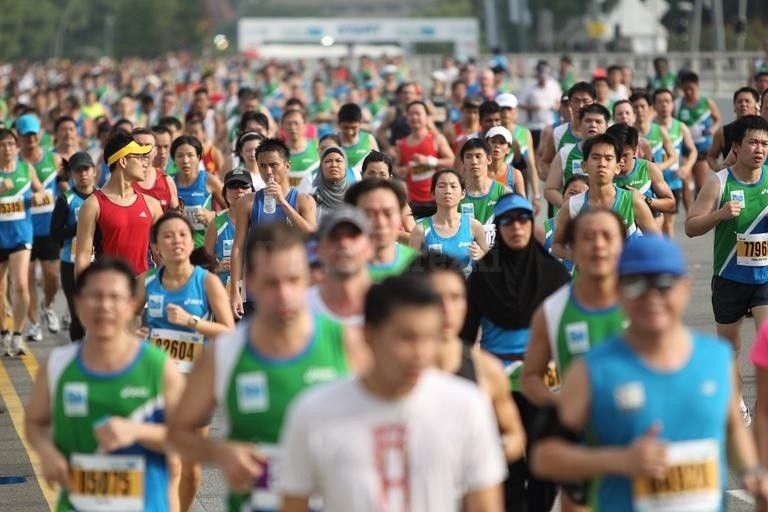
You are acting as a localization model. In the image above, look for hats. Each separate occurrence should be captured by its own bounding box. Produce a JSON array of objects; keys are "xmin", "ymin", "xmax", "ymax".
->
[
  {"xmin": 496, "ymin": 94, "xmax": 518, "ymax": 108},
  {"xmin": 224, "ymin": 169, "xmax": 252, "ymax": 187},
  {"xmin": 621, "ymin": 235, "xmax": 687, "ymax": 275},
  {"xmin": 108, "ymin": 141, "xmax": 152, "ymax": 166},
  {"xmin": 324, "ymin": 205, "xmax": 367, "ymax": 233},
  {"xmin": 16, "ymin": 114, "xmax": 41, "ymax": 135},
  {"xmin": 494, "ymin": 193, "xmax": 534, "ymax": 216},
  {"xmin": 485, "ymin": 126, "xmax": 513, "ymax": 146},
  {"xmin": 70, "ymin": 153, "xmax": 95, "ymax": 168}
]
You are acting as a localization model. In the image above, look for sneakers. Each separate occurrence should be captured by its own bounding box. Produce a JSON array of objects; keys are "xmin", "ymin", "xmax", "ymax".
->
[{"xmin": 2, "ymin": 304, "xmax": 73, "ymax": 357}]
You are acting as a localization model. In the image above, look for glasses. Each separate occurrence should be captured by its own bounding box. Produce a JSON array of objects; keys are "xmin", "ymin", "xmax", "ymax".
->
[
  {"xmin": 227, "ymin": 182, "xmax": 250, "ymax": 189},
  {"xmin": 498, "ymin": 212, "xmax": 530, "ymax": 224}
]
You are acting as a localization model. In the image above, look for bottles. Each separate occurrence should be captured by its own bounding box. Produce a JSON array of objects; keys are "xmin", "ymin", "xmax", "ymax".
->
[{"xmin": 262, "ymin": 177, "xmax": 276, "ymax": 214}]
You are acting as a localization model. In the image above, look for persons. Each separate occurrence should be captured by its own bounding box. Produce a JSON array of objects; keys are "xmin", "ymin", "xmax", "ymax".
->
[{"xmin": 1, "ymin": 44, "xmax": 768, "ymax": 511}]
